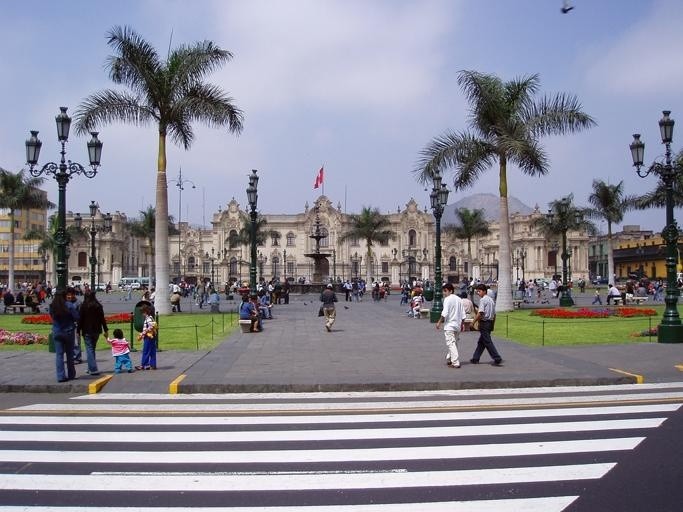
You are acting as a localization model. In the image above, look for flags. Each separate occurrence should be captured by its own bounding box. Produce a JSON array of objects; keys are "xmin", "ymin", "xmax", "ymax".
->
[{"xmin": 311, "ymin": 163, "xmax": 324, "ymax": 191}]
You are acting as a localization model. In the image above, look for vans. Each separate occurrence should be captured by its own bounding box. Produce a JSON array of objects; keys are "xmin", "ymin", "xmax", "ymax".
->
[
  {"xmin": 87, "ymin": 283, "xmax": 106, "ymax": 290},
  {"xmin": 676, "ymin": 273, "xmax": 682, "ymax": 284}
]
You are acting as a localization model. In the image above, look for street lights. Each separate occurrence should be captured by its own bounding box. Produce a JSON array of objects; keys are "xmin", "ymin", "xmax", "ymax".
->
[
  {"xmin": 635, "ymin": 244, "xmax": 643, "ymax": 281},
  {"xmin": 41, "ymin": 250, "xmax": 49, "ymax": 287},
  {"xmin": 245, "ymin": 170, "xmax": 257, "ymax": 307},
  {"xmin": 166, "ymin": 165, "xmax": 196, "ymax": 282},
  {"xmin": 24, "ymin": 106, "xmax": 103, "ymax": 353},
  {"xmin": 516, "ymin": 246, "xmax": 527, "ymax": 292},
  {"xmin": 628, "ymin": 111, "xmax": 682, "ymax": 343},
  {"xmin": 256, "ymin": 248, "xmax": 288, "ymax": 281},
  {"xmin": 205, "ymin": 246, "xmax": 220, "ymax": 294},
  {"xmin": 428, "ymin": 169, "xmax": 450, "ymax": 323},
  {"xmin": 73, "ymin": 200, "xmax": 112, "ymax": 299},
  {"xmin": 402, "ymin": 246, "xmax": 417, "ymax": 287},
  {"xmin": 545, "ymin": 198, "xmax": 582, "ymax": 307},
  {"xmin": 331, "ymin": 248, "xmax": 336, "ymax": 281},
  {"xmin": 349, "ymin": 251, "xmax": 362, "ymax": 281},
  {"xmin": 95, "ymin": 254, "xmax": 104, "ymax": 292}
]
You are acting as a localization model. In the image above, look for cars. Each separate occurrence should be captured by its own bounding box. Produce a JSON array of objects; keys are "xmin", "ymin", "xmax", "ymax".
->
[
  {"xmin": 400, "ymin": 280, "xmax": 426, "ymax": 289},
  {"xmin": 591, "ymin": 276, "xmax": 608, "ymax": 285},
  {"xmin": 489, "ymin": 280, "xmax": 497, "ymax": 286},
  {"xmin": 528, "ymin": 278, "xmax": 550, "ymax": 287},
  {"xmin": 364, "ymin": 280, "xmax": 391, "ymax": 290}
]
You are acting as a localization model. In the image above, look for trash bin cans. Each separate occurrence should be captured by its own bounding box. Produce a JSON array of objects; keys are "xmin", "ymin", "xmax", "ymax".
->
[
  {"xmin": 134, "ymin": 300, "xmax": 155, "ymax": 332},
  {"xmin": 424, "ymin": 287, "xmax": 433, "ymax": 301}
]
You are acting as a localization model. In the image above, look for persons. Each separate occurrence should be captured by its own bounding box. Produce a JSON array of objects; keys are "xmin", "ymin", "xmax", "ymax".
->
[
  {"xmin": 515, "ymin": 277, "xmax": 683, "ymax": 306},
  {"xmin": 340, "ymin": 270, "xmax": 495, "ymax": 318},
  {"xmin": 63, "ymin": 286, "xmax": 82, "ymax": 364},
  {"xmin": 0, "ymin": 275, "xmax": 290, "ymax": 333},
  {"xmin": 434, "ymin": 282, "xmax": 467, "ymax": 368},
  {"xmin": 316, "ymin": 283, "xmax": 338, "ymax": 332},
  {"xmin": 74, "ymin": 290, "xmax": 108, "ymax": 374},
  {"xmin": 103, "ymin": 327, "xmax": 132, "ymax": 374},
  {"xmin": 468, "ymin": 284, "xmax": 502, "ymax": 367},
  {"xmin": 47, "ymin": 287, "xmax": 80, "ymax": 383},
  {"xmin": 133, "ymin": 304, "xmax": 158, "ymax": 371}
]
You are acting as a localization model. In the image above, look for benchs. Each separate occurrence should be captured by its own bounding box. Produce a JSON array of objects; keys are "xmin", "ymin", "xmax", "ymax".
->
[
  {"xmin": 613, "ymin": 297, "xmax": 648, "ymax": 305},
  {"xmin": 418, "ymin": 309, "xmax": 429, "ymax": 319},
  {"xmin": 513, "ymin": 300, "xmax": 524, "ymax": 308},
  {"xmin": 239, "ymin": 319, "xmax": 253, "ymax": 333},
  {"xmin": 463, "ymin": 318, "xmax": 474, "ymax": 331}
]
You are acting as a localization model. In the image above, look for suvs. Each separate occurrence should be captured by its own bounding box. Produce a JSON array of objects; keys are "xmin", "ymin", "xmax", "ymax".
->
[{"xmin": 125, "ymin": 282, "xmax": 141, "ymax": 291}]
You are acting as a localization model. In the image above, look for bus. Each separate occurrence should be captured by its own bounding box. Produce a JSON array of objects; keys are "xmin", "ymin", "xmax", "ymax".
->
[
  {"xmin": 116, "ymin": 276, "xmax": 154, "ymax": 290},
  {"xmin": 172, "ymin": 277, "xmax": 210, "ymax": 291}
]
